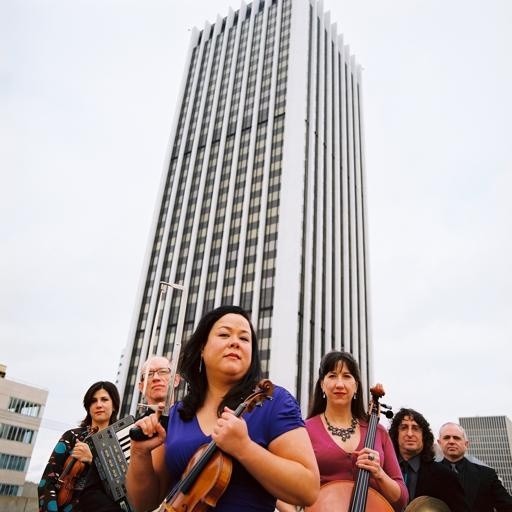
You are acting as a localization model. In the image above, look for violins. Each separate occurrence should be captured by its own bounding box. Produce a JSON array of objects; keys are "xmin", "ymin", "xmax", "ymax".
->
[
  {"xmin": 155, "ymin": 381, "xmax": 272, "ymax": 512},
  {"xmin": 54, "ymin": 426, "xmax": 100, "ymax": 505}
]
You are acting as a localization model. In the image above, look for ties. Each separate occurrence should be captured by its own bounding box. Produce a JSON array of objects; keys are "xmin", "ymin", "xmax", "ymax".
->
[
  {"xmin": 451, "ymin": 463, "xmax": 458, "ymax": 476},
  {"xmin": 402, "ymin": 461, "xmax": 412, "ymax": 489}
]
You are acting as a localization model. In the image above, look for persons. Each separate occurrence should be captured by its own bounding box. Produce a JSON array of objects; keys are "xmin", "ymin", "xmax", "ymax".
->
[
  {"xmin": 435, "ymin": 422, "xmax": 511, "ymax": 511},
  {"xmin": 124, "ymin": 306, "xmax": 320, "ymax": 511},
  {"xmin": 132, "ymin": 357, "xmax": 179, "ymax": 431},
  {"xmin": 387, "ymin": 408, "xmax": 471, "ymax": 512},
  {"xmin": 36, "ymin": 380, "xmax": 126, "ymax": 511},
  {"xmin": 273, "ymin": 351, "xmax": 409, "ymax": 511}
]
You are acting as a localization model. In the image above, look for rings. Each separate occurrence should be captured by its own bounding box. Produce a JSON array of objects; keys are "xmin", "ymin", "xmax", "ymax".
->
[{"xmin": 366, "ymin": 454, "xmax": 373, "ymax": 461}]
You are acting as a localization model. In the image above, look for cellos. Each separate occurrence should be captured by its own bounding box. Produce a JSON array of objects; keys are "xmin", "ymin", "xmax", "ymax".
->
[{"xmin": 301, "ymin": 383, "xmax": 398, "ymax": 512}]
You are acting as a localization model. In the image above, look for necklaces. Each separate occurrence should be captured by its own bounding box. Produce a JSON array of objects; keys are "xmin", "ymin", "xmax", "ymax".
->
[{"xmin": 322, "ymin": 413, "xmax": 359, "ymax": 441}]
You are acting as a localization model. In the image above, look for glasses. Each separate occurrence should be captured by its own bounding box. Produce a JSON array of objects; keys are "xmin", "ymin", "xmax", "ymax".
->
[{"xmin": 141, "ymin": 367, "xmax": 172, "ymax": 382}]
